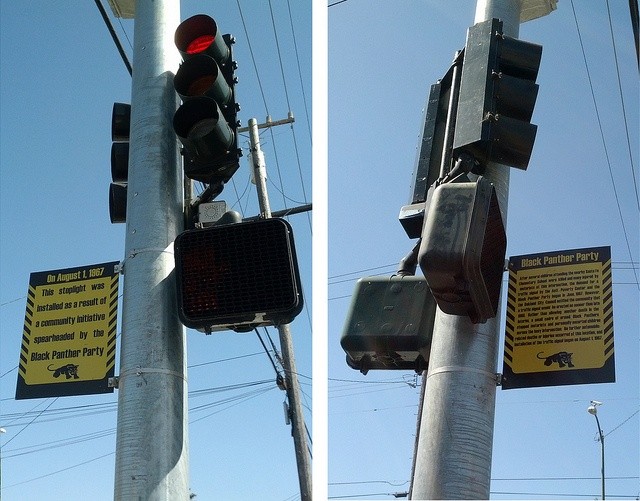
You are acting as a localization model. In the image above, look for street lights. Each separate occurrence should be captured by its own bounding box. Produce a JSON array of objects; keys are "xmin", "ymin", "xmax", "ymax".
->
[{"xmin": 588, "ymin": 400, "xmax": 604, "ymax": 500}]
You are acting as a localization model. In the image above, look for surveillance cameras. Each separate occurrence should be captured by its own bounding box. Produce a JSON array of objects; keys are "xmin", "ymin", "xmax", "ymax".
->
[{"xmin": 590, "ymin": 401, "xmax": 602, "ymax": 407}]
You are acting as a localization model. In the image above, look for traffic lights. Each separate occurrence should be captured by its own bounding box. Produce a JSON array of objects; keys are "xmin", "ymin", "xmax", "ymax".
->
[
  {"xmin": 173, "ymin": 13, "xmax": 241, "ymax": 184},
  {"xmin": 417, "ymin": 174, "xmax": 507, "ymax": 323},
  {"xmin": 451, "ymin": 18, "xmax": 542, "ymax": 169},
  {"xmin": 109, "ymin": 103, "xmax": 130, "ymax": 223},
  {"xmin": 173, "ymin": 217, "xmax": 302, "ymax": 335}
]
